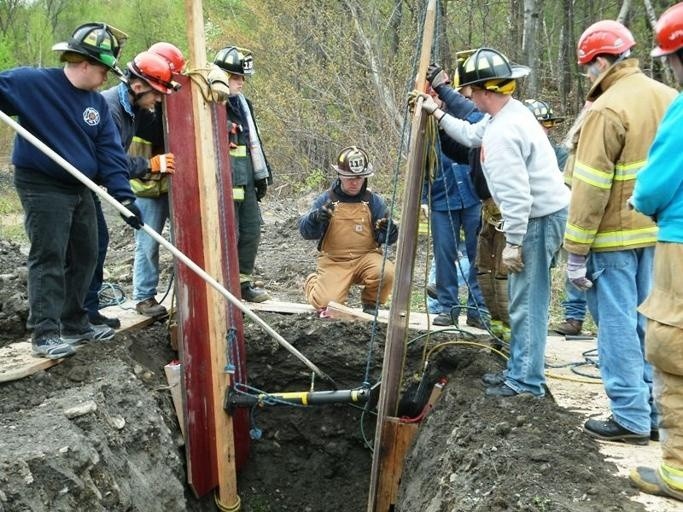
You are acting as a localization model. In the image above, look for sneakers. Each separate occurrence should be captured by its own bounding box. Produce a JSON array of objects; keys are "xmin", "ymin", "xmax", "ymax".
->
[
  {"xmin": 484, "ymin": 383, "xmax": 518, "ymax": 399},
  {"xmin": 362, "ymin": 302, "xmax": 378, "ymax": 317},
  {"xmin": 648, "ymin": 430, "xmax": 659, "ymax": 442},
  {"xmin": 89, "ymin": 313, "xmax": 120, "ymax": 329},
  {"xmin": 480, "ymin": 373, "xmax": 507, "ymax": 388},
  {"xmin": 555, "ymin": 319, "xmax": 583, "ymax": 335},
  {"xmin": 465, "ymin": 317, "xmax": 490, "ymax": 330},
  {"xmin": 627, "ymin": 466, "xmax": 664, "ymax": 496},
  {"xmin": 432, "ymin": 312, "xmax": 459, "ymax": 326},
  {"xmin": 240, "ymin": 281, "xmax": 270, "ymax": 303},
  {"xmin": 31, "ymin": 333, "xmax": 77, "ymax": 360},
  {"xmin": 61, "ymin": 326, "xmax": 116, "ymax": 341},
  {"xmin": 583, "ymin": 418, "xmax": 650, "ymax": 446},
  {"xmin": 136, "ymin": 296, "xmax": 167, "ymax": 317}
]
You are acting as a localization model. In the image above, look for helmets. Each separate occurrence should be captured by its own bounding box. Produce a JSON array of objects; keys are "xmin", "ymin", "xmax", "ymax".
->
[
  {"xmin": 213, "ymin": 46, "xmax": 255, "ymax": 77},
  {"xmin": 50, "ymin": 21, "xmax": 129, "ymax": 77},
  {"xmin": 330, "ymin": 145, "xmax": 374, "ymax": 177},
  {"xmin": 521, "ymin": 98, "xmax": 566, "ymax": 128},
  {"xmin": 576, "ymin": 19, "xmax": 637, "ymax": 64},
  {"xmin": 126, "ymin": 50, "xmax": 172, "ymax": 95},
  {"xmin": 147, "ymin": 41, "xmax": 186, "ymax": 72},
  {"xmin": 454, "ymin": 47, "xmax": 531, "ymax": 94},
  {"xmin": 649, "ymin": 1, "xmax": 683, "ymax": 57}
]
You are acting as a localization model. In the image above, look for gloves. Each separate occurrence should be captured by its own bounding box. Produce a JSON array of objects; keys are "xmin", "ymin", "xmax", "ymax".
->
[
  {"xmin": 407, "ymin": 89, "xmax": 439, "ymax": 115},
  {"xmin": 119, "ymin": 203, "xmax": 145, "ymax": 230},
  {"xmin": 378, "ymin": 218, "xmax": 397, "ymax": 236},
  {"xmin": 150, "ymin": 152, "xmax": 176, "ymax": 175},
  {"xmin": 567, "ymin": 252, "xmax": 593, "ymax": 293},
  {"xmin": 255, "ymin": 178, "xmax": 267, "ymax": 203},
  {"xmin": 425, "ymin": 62, "xmax": 451, "ymax": 93},
  {"xmin": 501, "ymin": 242, "xmax": 525, "ymax": 273},
  {"xmin": 318, "ymin": 205, "xmax": 333, "ymax": 224}
]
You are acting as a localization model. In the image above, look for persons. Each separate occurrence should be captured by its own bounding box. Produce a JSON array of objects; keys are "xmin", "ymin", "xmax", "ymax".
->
[
  {"xmin": 83, "ymin": 51, "xmax": 183, "ymax": 330},
  {"xmin": 415, "ymin": 47, "xmax": 570, "ymax": 398},
  {"xmin": 561, "ymin": 20, "xmax": 680, "ymax": 447},
  {"xmin": 127, "ymin": 41, "xmax": 188, "ymax": 318},
  {"xmin": 299, "ymin": 144, "xmax": 399, "ymax": 317},
  {"xmin": 0, "ymin": 21, "xmax": 144, "ymax": 360},
  {"xmin": 210, "ymin": 45, "xmax": 274, "ymax": 302},
  {"xmin": 424, "ymin": 59, "xmax": 510, "ymax": 356},
  {"xmin": 518, "ymin": 96, "xmax": 568, "ymax": 172},
  {"xmin": 405, "ymin": 72, "xmax": 493, "ymax": 331},
  {"xmin": 550, "ymin": 97, "xmax": 600, "ymax": 338},
  {"xmin": 627, "ymin": 0, "xmax": 683, "ymax": 505}
]
